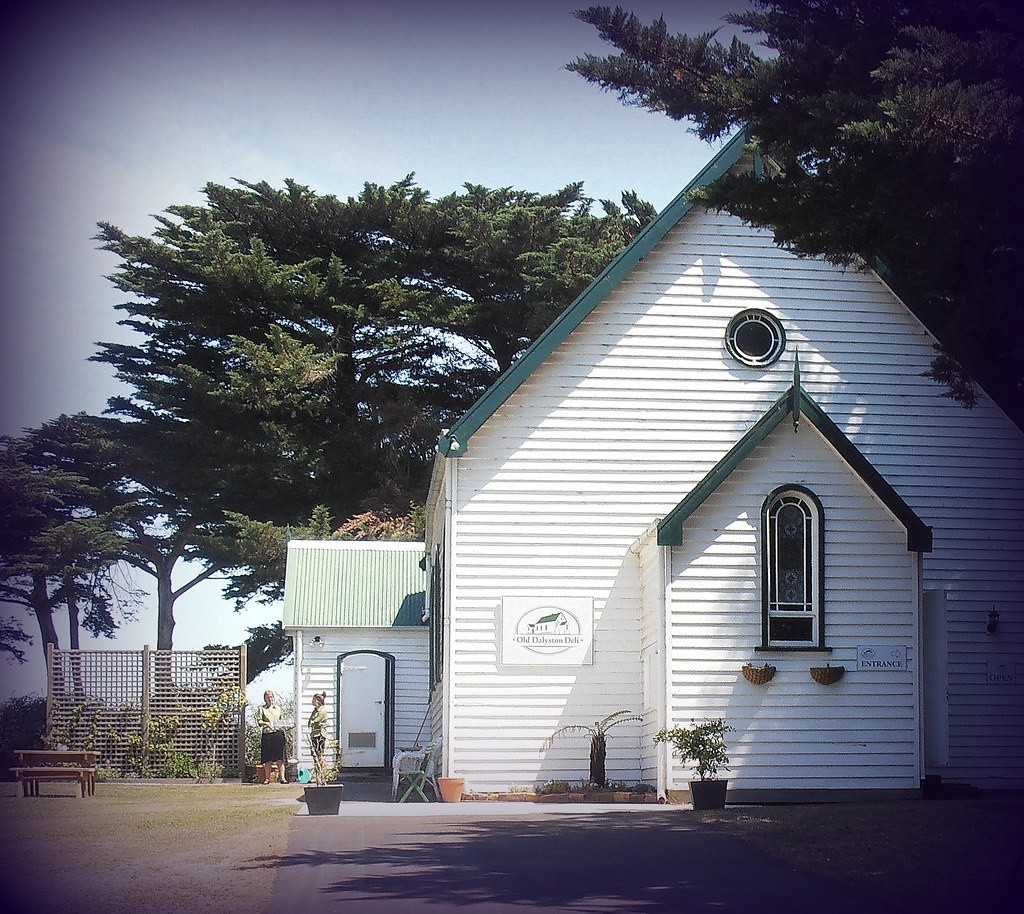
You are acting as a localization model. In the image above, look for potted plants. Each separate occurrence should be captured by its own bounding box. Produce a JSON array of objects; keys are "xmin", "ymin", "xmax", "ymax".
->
[
  {"xmin": 301, "ymin": 729, "xmax": 345, "ymax": 816},
  {"xmin": 657, "ymin": 717, "xmax": 734, "ymax": 811}
]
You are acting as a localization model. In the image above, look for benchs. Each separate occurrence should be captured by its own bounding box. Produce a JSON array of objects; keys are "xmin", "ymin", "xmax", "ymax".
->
[{"xmin": 8, "ymin": 767, "xmax": 96, "ymax": 798}]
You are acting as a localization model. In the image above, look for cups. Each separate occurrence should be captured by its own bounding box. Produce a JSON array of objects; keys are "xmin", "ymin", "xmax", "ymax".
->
[{"xmin": 269, "ymin": 718, "xmax": 294, "ymax": 729}]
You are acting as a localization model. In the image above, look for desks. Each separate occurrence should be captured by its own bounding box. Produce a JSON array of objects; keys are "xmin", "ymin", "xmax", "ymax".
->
[{"xmin": 13, "ymin": 748, "xmax": 100, "ymax": 791}]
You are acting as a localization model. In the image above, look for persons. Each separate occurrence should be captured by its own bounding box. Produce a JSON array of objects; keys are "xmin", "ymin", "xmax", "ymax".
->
[
  {"xmin": 307, "ymin": 691, "xmax": 330, "ymax": 782},
  {"xmin": 257, "ymin": 690, "xmax": 289, "ymax": 785}
]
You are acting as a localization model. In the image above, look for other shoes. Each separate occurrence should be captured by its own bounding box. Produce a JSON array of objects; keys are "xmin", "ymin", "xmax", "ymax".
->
[
  {"xmin": 281, "ymin": 780, "xmax": 289, "ymax": 784},
  {"xmin": 263, "ymin": 781, "xmax": 269, "ymax": 784}
]
